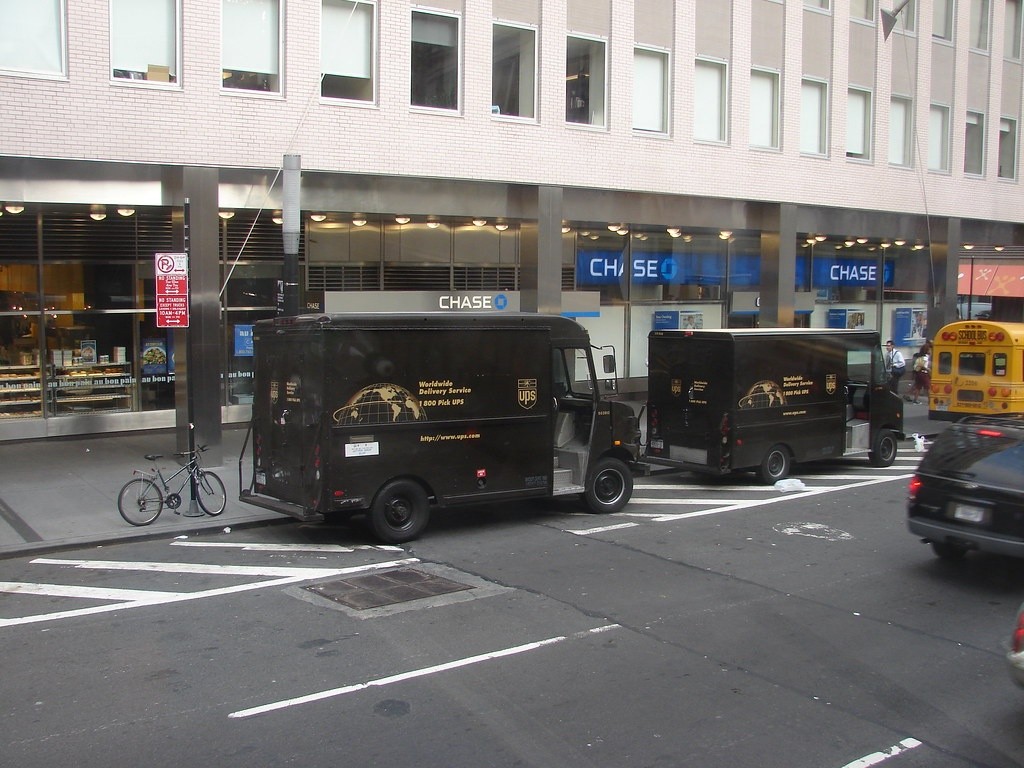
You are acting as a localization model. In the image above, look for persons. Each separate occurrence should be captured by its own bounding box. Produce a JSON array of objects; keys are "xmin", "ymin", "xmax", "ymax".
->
[
  {"xmin": 855, "ymin": 314, "xmax": 864, "ymax": 329},
  {"xmin": 686, "ymin": 316, "xmax": 697, "ymax": 329},
  {"xmin": 903, "ymin": 345, "xmax": 932, "ymax": 405},
  {"xmin": 886, "ymin": 341, "xmax": 905, "ymax": 394}
]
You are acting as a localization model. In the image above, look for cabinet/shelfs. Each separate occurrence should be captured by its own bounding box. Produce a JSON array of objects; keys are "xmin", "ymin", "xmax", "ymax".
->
[{"xmin": 0, "ymin": 362, "xmax": 135, "ymax": 420}]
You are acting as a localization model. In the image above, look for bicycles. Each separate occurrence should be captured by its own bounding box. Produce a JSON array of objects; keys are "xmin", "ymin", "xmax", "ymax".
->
[{"xmin": 117, "ymin": 444, "xmax": 227, "ymax": 526}]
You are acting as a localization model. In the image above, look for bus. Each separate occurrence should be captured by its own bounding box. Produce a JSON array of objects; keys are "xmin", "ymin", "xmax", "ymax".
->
[{"xmin": 927, "ymin": 319, "xmax": 1024, "ymax": 425}]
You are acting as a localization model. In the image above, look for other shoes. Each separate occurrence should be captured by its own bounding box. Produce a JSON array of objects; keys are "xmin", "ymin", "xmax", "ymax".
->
[
  {"xmin": 913, "ymin": 400, "xmax": 922, "ymax": 405},
  {"xmin": 903, "ymin": 395, "xmax": 912, "ymax": 402}
]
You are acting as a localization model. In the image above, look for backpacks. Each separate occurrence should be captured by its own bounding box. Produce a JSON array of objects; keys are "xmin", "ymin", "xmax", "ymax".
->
[{"xmin": 913, "ymin": 353, "xmax": 926, "ymax": 372}]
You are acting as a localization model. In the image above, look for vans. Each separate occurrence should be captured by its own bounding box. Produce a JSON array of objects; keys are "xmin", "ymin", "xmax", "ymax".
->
[
  {"xmin": 237, "ymin": 311, "xmax": 651, "ymax": 547},
  {"xmin": 639, "ymin": 327, "xmax": 907, "ymax": 486}
]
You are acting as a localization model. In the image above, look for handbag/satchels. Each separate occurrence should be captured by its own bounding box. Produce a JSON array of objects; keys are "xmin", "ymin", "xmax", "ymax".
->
[{"xmin": 892, "ymin": 366, "xmax": 905, "ymax": 376}]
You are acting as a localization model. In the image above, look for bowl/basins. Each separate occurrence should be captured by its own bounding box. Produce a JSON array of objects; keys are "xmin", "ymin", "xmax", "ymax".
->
[{"xmin": 82, "ymin": 351, "xmax": 95, "ymax": 359}]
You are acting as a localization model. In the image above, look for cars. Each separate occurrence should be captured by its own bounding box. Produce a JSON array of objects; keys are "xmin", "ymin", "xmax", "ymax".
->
[{"xmin": 905, "ymin": 412, "xmax": 1024, "ymax": 558}]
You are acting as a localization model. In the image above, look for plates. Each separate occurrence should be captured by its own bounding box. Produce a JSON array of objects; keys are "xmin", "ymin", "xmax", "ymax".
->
[{"xmin": 143, "ymin": 347, "xmax": 166, "ymax": 363}]
